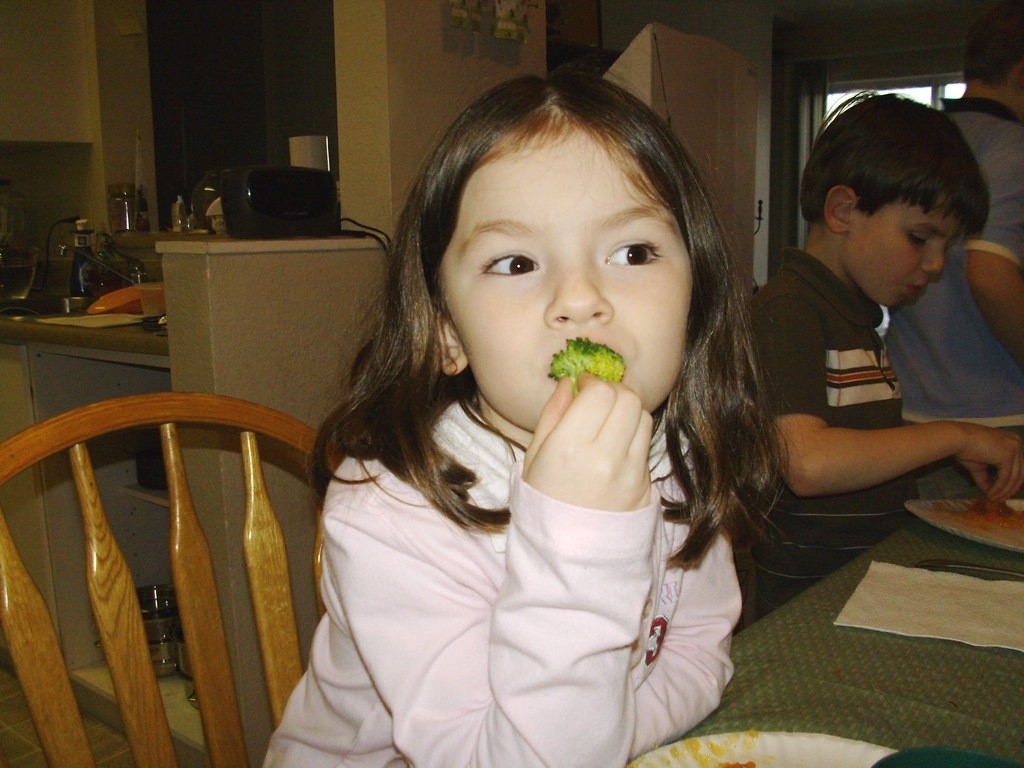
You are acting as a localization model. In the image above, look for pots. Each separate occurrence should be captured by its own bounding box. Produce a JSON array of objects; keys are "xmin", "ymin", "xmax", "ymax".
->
[{"xmin": 97, "ymin": 584, "xmax": 199, "ymax": 709}]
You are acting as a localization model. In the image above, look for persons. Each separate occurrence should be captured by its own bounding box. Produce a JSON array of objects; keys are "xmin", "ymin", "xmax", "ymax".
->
[
  {"xmin": 262, "ymin": 69, "xmax": 799, "ymax": 767},
  {"xmin": 745, "ymin": 1, "xmax": 1024, "ymax": 621}
]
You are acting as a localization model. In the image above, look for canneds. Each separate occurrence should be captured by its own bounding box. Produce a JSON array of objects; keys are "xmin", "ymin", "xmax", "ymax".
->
[{"xmin": 107, "ymin": 182, "xmax": 137, "ymax": 232}]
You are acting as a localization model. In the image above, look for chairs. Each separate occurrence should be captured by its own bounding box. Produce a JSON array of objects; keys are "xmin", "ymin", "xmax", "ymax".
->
[{"xmin": 0, "ymin": 391, "xmax": 341, "ymax": 768}]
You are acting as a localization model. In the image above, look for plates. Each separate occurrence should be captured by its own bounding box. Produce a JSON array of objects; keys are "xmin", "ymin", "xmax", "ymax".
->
[
  {"xmin": 905, "ymin": 495, "xmax": 1024, "ymax": 554},
  {"xmin": 625, "ymin": 730, "xmax": 900, "ymax": 768}
]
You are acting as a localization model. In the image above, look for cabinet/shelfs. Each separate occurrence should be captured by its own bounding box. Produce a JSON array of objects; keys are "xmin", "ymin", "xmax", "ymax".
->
[{"xmin": 2, "ymin": 315, "xmax": 202, "ymax": 763}]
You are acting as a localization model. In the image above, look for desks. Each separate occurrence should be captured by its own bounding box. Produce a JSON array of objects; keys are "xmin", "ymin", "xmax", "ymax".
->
[{"xmin": 679, "ymin": 518, "xmax": 1024, "ymax": 767}]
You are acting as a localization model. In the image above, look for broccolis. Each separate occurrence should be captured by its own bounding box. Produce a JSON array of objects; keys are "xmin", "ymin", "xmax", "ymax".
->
[{"xmin": 549, "ymin": 336, "xmax": 625, "ymax": 400}]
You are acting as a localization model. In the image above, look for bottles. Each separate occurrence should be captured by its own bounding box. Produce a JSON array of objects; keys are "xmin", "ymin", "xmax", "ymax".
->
[{"xmin": 107, "ymin": 183, "xmax": 138, "ymax": 233}]
